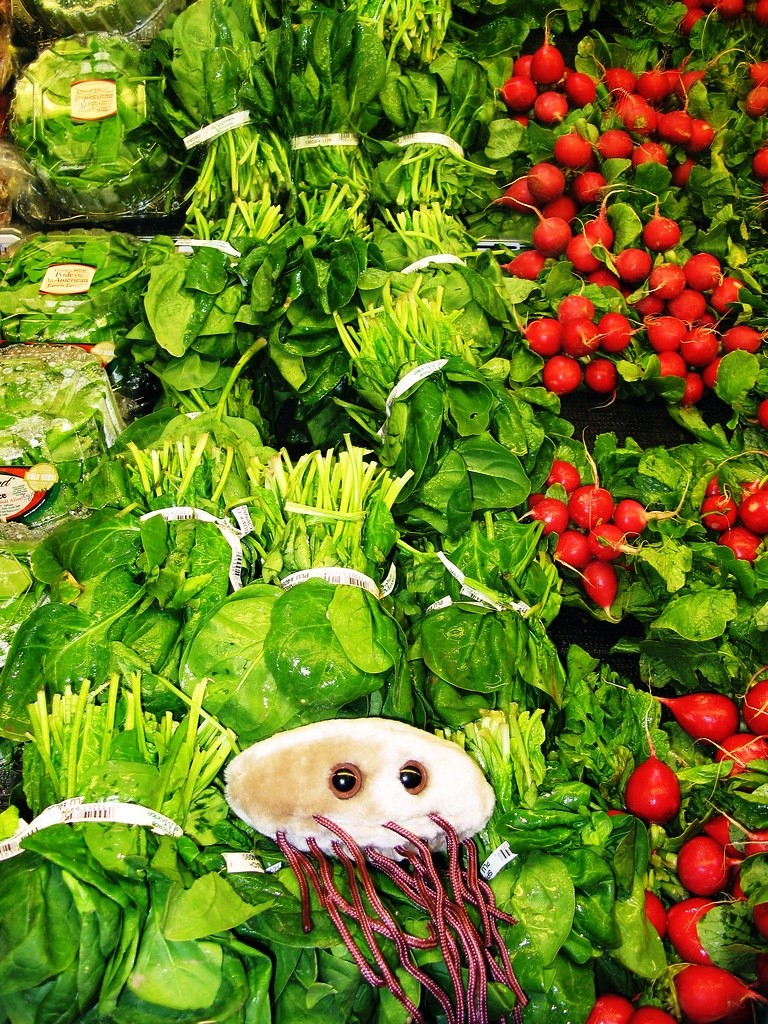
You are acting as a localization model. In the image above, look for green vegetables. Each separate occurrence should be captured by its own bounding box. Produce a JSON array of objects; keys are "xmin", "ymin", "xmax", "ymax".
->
[{"xmin": 0, "ymin": 0, "xmax": 668, "ymax": 1024}]
